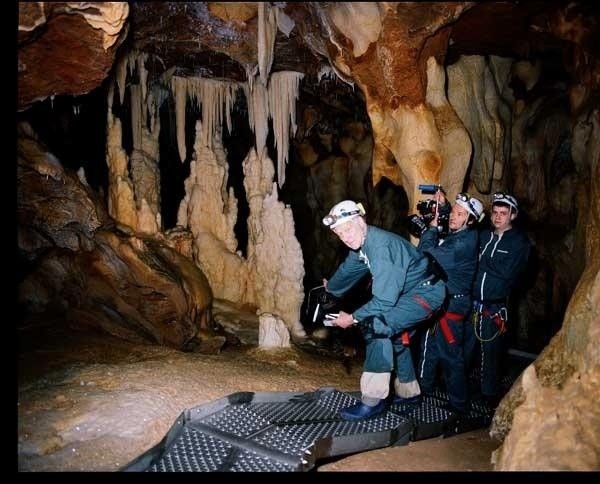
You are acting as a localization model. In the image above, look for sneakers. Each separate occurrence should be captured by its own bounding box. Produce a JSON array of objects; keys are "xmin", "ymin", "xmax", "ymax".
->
[
  {"xmin": 339, "ymin": 399, "xmax": 386, "ymax": 421},
  {"xmin": 386, "ymin": 393, "xmax": 420, "ymax": 405}
]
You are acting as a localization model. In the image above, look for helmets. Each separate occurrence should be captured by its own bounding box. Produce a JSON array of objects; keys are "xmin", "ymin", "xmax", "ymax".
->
[
  {"xmin": 322, "ymin": 200, "xmax": 366, "ymax": 229},
  {"xmin": 492, "ymin": 192, "xmax": 519, "ymax": 214},
  {"xmin": 455, "ymin": 197, "xmax": 484, "ymax": 221}
]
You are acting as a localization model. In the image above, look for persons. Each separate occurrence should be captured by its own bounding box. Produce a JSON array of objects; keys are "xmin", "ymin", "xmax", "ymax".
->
[
  {"xmin": 460, "ymin": 191, "xmax": 533, "ymax": 407},
  {"xmin": 320, "ymin": 199, "xmax": 450, "ymax": 422},
  {"xmin": 415, "ymin": 188, "xmax": 484, "ymax": 412}
]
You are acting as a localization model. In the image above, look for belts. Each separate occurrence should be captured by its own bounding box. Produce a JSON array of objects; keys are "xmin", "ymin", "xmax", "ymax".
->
[
  {"xmin": 421, "ymin": 276, "xmax": 440, "ymax": 286},
  {"xmin": 447, "ymin": 293, "xmax": 468, "ymax": 298},
  {"xmin": 484, "ymin": 298, "xmax": 505, "ymax": 304}
]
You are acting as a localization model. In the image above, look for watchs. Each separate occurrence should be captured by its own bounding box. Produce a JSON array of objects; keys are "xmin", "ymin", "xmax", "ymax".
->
[{"xmin": 351, "ymin": 312, "xmax": 360, "ymax": 324}]
